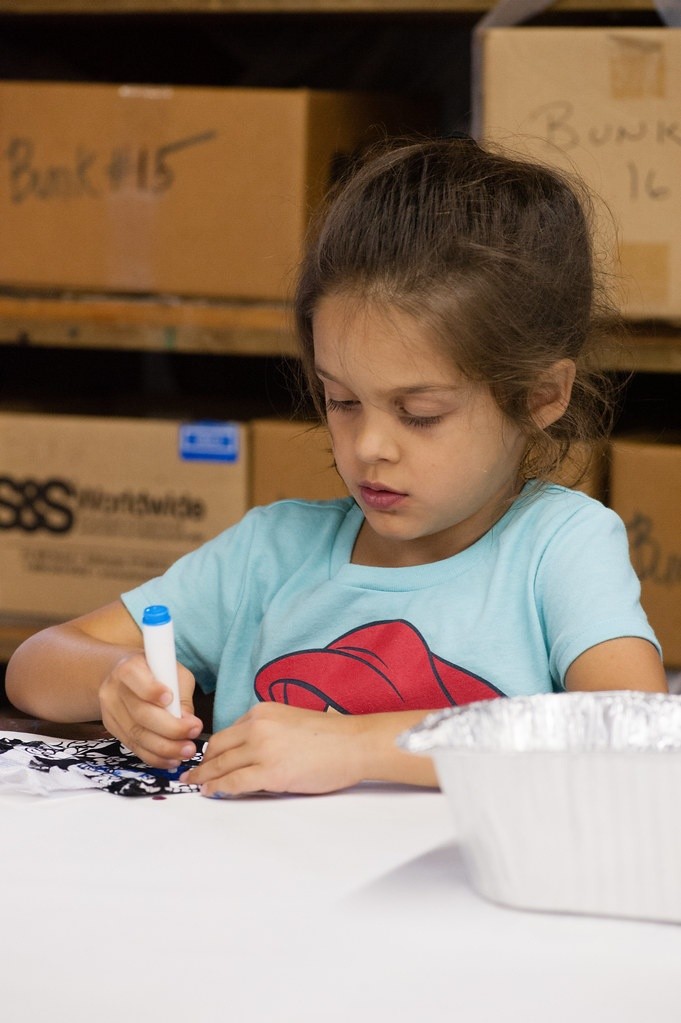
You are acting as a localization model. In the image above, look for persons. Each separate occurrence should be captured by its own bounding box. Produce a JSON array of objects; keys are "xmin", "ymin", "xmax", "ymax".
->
[{"xmin": 5, "ymin": 140, "xmax": 667, "ymax": 798}]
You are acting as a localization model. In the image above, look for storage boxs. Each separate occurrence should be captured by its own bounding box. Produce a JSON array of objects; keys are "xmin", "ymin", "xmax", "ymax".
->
[
  {"xmin": 0, "ymin": 416, "xmax": 681, "ymax": 676},
  {"xmin": 468, "ymin": 1, "xmax": 681, "ymax": 323},
  {"xmin": 1, "ymin": 81, "xmax": 383, "ymax": 355}
]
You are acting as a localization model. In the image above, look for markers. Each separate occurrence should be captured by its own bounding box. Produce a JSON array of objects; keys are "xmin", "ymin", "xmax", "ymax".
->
[{"xmin": 141, "ymin": 604, "xmax": 184, "ymax": 775}]
